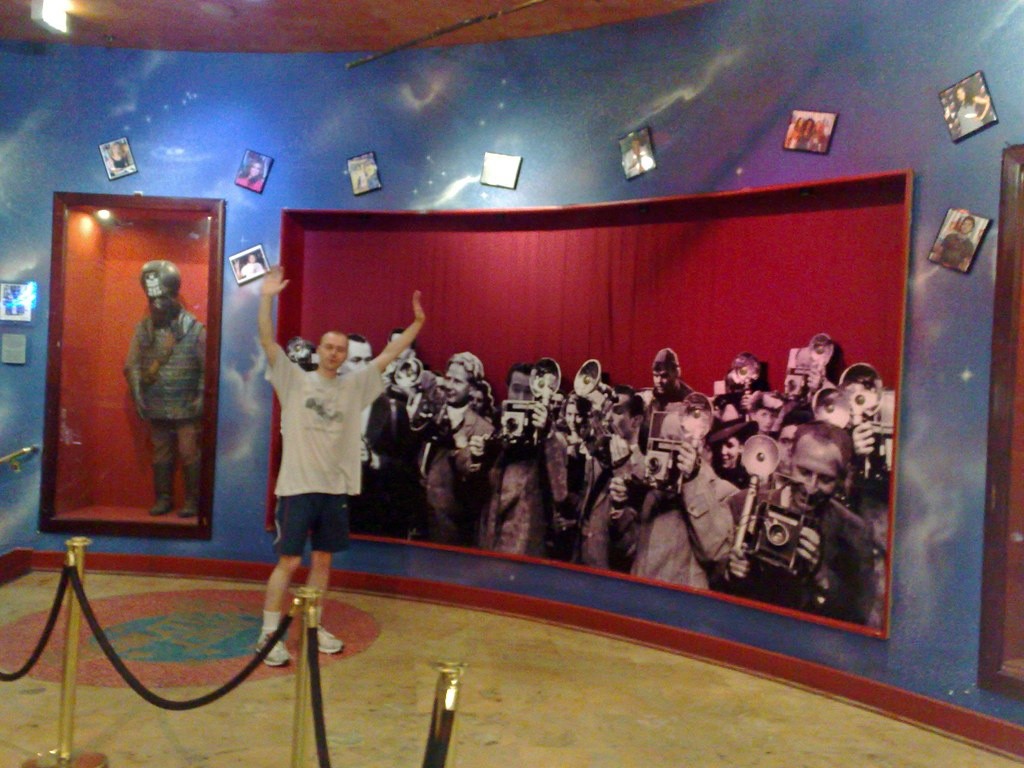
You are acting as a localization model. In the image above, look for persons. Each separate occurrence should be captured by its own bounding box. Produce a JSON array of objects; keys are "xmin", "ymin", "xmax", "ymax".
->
[
  {"xmin": 233, "ymin": 253, "xmax": 267, "ymax": 279},
  {"xmin": 235, "ymin": 158, "xmax": 265, "ymax": 192},
  {"xmin": 349, "ymin": 152, "xmax": 382, "ymax": 193},
  {"xmin": 106, "ymin": 142, "xmax": 133, "ymax": 176},
  {"xmin": 930, "ymin": 216, "xmax": 976, "ymax": 271},
  {"xmin": 623, "ymin": 137, "xmax": 655, "ymax": 175},
  {"xmin": 944, "ymin": 84, "xmax": 991, "ymax": 137},
  {"xmin": 256, "ymin": 265, "xmax": 425, "ymax": 670},
  {"xmin": 124, "ymin": 261, "xmax": 207, "ymax": 518},
  {"xmin": 284, "ymin": 327, "xmax": 895, "ymax": 625},
  {"xmin": 784, "ymin": 117, "xmax": 828, "ymax": 154}
]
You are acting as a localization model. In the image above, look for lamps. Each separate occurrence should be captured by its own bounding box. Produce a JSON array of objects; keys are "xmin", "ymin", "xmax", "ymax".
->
[{"xmin": 29, "ymin": 0, "xmax": 71, "ymax": 35}]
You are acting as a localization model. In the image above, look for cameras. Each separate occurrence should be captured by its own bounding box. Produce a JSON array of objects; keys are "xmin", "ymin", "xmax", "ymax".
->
[{"xmin": 287, "ymin": 333, "xmax": 893, "ymax": 577}]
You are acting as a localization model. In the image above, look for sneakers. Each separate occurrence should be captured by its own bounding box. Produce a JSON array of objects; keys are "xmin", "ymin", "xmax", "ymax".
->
[
  {"xmin": 254, "ymin": 629, "xmax": 291, "ymax": 665},
  {"xmin": 315, "ymin": 625, "xmax": 344, "ymax": 653}
]
